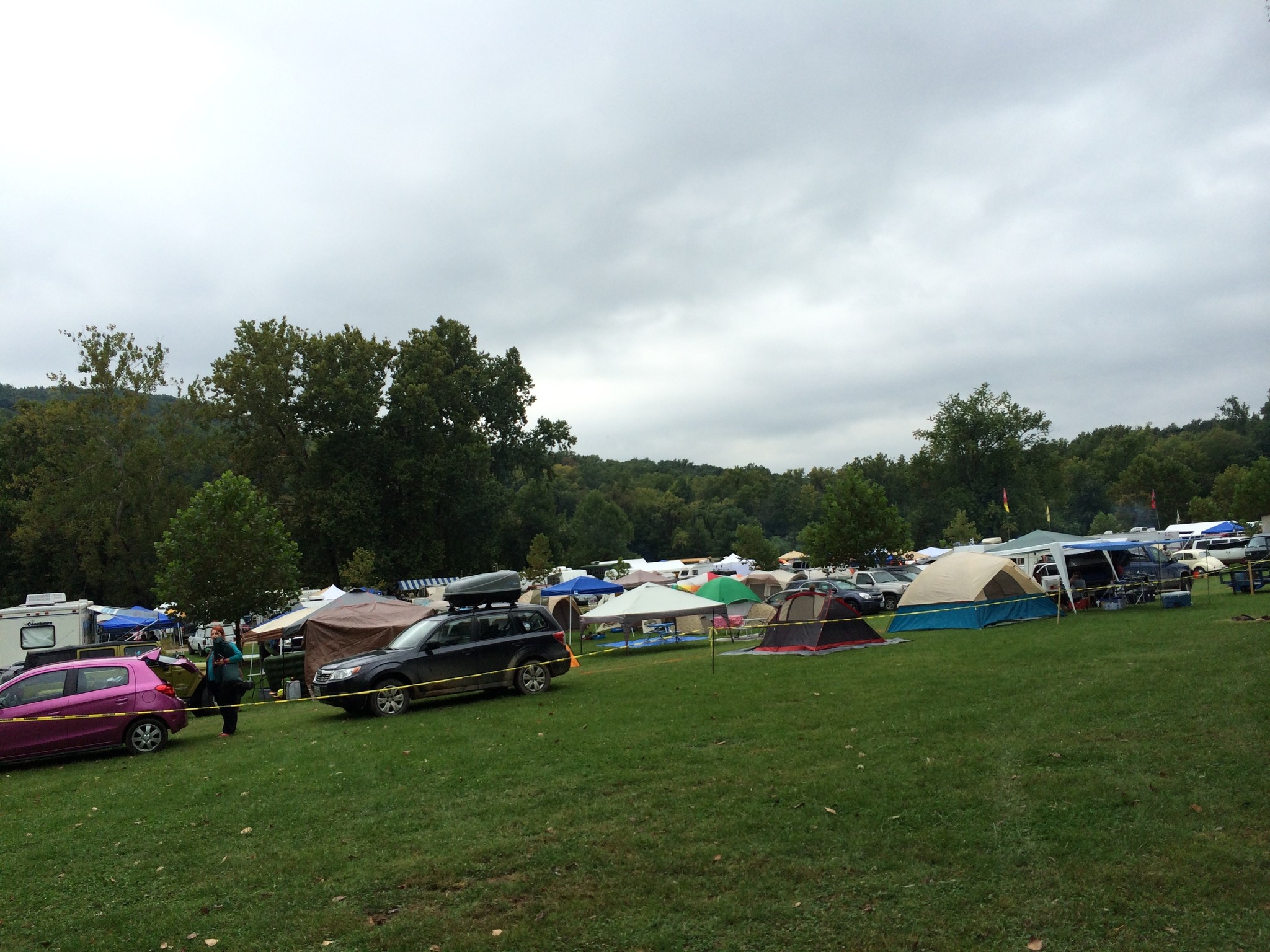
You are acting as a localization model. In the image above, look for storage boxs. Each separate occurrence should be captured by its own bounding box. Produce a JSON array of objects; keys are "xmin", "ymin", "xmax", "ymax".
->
[
  {"xmin": 1161, "ymin": 591, "xmax": 1191, "ymax": 607},
  {"xmin": 1100, "ymin": 599, "xmax": 1126, "ymax": 608}
]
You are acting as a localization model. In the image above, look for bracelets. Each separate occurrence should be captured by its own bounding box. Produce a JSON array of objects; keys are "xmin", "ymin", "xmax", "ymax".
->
[{"xmin": 224, "ymin": 658, "xmax": 227, "ymax": 666}]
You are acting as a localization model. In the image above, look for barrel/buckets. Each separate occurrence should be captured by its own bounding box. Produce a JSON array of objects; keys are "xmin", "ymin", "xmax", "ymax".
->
[{"xmin": 1194, "ymin": 572, "xmax": 1199, "ymax": 578}]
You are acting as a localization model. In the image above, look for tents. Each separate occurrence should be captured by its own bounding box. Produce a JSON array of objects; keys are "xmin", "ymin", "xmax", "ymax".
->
[
  {"xmin": 98, "ymin": 518, "xmax": 1248, "ymax": 651},
  {"xmin": 577, "ymin": 581, "xmax": 736, "ymax": 674},
  {"xmin": 241, "ymin": 591, "xmax": 438, "ymax": 701},
  {"xmin": 749, "ymin": 588, "xmax": 888, "ymax": 651}
]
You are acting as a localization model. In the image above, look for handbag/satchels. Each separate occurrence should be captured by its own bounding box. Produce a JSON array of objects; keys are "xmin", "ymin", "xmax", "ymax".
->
[{"xmin": 263, "ymin": 640, "xmax": 278, "ymax": 654}]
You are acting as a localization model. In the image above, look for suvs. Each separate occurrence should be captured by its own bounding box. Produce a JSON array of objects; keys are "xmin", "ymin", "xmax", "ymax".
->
[
  {"xmin": 258, "ymin": 613, "xmax": 304, "ymax": 668},
  {"xmin": 174, "ymin": 622, "xmax": 237, "ymax": 657},
  {"xmin": 783, "ymin": 578, "xmax": 885, "ymax": 616},
  {"xmin": 1018, "ymin": 563, "xmax": 1065, "ymax": 590},
  {"xmin": 822, "ymin": 565, "xmax": 923, "ymax": 611},
  {"xmin": 23, "ymin": 641, "xmax": 253, "ymax": 718},
  {"xmin": 310, "ymin": 570, "xmax": 571, "ymax": 717}
]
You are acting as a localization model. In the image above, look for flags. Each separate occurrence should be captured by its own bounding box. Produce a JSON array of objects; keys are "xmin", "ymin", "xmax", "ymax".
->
[
  {"xmin": 1176, "ymin": 510, "xmax": 1180, "ymax": 524},
  {"xmin": 1151, "ymin": 489, "xmax": 1156, "ymax": 509},
  {"xmin": 1046, "ymin": 505, "xmax": 1050, "ymax": 522},
  {"xmin": 1003, "ymin": 488, "xmax": 1009, "ymax": 512}
]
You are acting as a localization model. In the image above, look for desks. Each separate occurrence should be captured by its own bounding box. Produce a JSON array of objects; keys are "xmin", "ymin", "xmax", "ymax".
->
[
  {"xmin": 646, "ymin": 621, "xmax": 675, "ymax": 630},
  {"xmin": 740, "ymin": 616, "xmax": 770, "ymax": 640}
]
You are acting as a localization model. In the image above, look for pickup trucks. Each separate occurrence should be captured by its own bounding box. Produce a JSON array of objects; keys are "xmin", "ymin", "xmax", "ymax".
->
[{"xmin": 1064, "ymin": 545, "xmax": 1195, "ymax": 604}]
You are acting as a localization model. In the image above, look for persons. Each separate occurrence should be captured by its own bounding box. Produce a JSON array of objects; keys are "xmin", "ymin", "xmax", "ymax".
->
[
  {"xmin": 206, "ymin": 626, "xmax": 244, "ymax": 736},
  {"xmin": 1062, "ymin": 572, "xmax": 1086, "ymax": 603},
  {"xmin": 1111, "ymin": 561, "xmax": 1124, "ymax": 580},
  {"xmin": 127, "ymin": 634, "xmax": 136, "ymax": 641},
  {"xmin": 1159, "ymin": 545, "xmax": 1173, "ymax": 557},
  {"xmin": 141, "ymin": 634, "xmax": 148, "ymax": 641},
  {"xmin": 149, "ymin": 631, "xmax": 159, "ymax": 641}
]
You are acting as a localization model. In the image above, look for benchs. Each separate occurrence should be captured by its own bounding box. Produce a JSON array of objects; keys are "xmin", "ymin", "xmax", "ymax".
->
[
  {"xmin": 732, "ymin": 627, "xmax": 751, "ymax": 631},
  {"xmin": 643, "ymin": 630, "xmax": 682, "ymax": 641}
]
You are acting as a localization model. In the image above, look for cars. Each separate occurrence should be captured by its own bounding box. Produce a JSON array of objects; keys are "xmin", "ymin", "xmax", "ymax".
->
[
  {"xmin": 1169, "ymin": 549, "xmax": 1228, "ymax": 576},
  {"xmin": 0, "ymin": 647, "xmax": 206, "ymax": 763},
  {"xmin": 761, "ymin": 588, "xmax": 844, "ymax": 609}
]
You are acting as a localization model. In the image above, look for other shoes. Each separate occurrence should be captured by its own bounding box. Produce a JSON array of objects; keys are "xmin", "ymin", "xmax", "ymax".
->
[{"xmin": 217, "ymin": 732, "xmax": 229, "ymax": 737}]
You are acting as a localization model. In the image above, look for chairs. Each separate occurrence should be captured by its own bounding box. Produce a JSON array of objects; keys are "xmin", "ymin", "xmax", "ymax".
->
[
  {"xmin": 827, "ymin": 583, "xmax": 833, "ymax": 589},
  {"xmin": 248, "ymin": 664, "xmax": 271, "ymax": 699},
  {"xmin": 77, "ymin": 671, "xmax": 88, "ymax": 694},
  {"xmin": 1071, "ymin": 589, "xmax": 1088, "ymax": 610},
  {"xmin": 443, "ymin": 616, "xmax": 512, "ymax": 644}
]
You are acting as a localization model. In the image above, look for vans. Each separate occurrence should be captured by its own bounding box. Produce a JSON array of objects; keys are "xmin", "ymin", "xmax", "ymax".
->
[{"xmin": 1193, "ymin": 533, "xmax": 1270, "ymax": 566}]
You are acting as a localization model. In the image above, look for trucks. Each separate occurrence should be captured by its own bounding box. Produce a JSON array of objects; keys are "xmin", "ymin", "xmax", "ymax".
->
[{"xmin": 0, "ymin": 592, "xmax": 104, "ymax": 677}]
